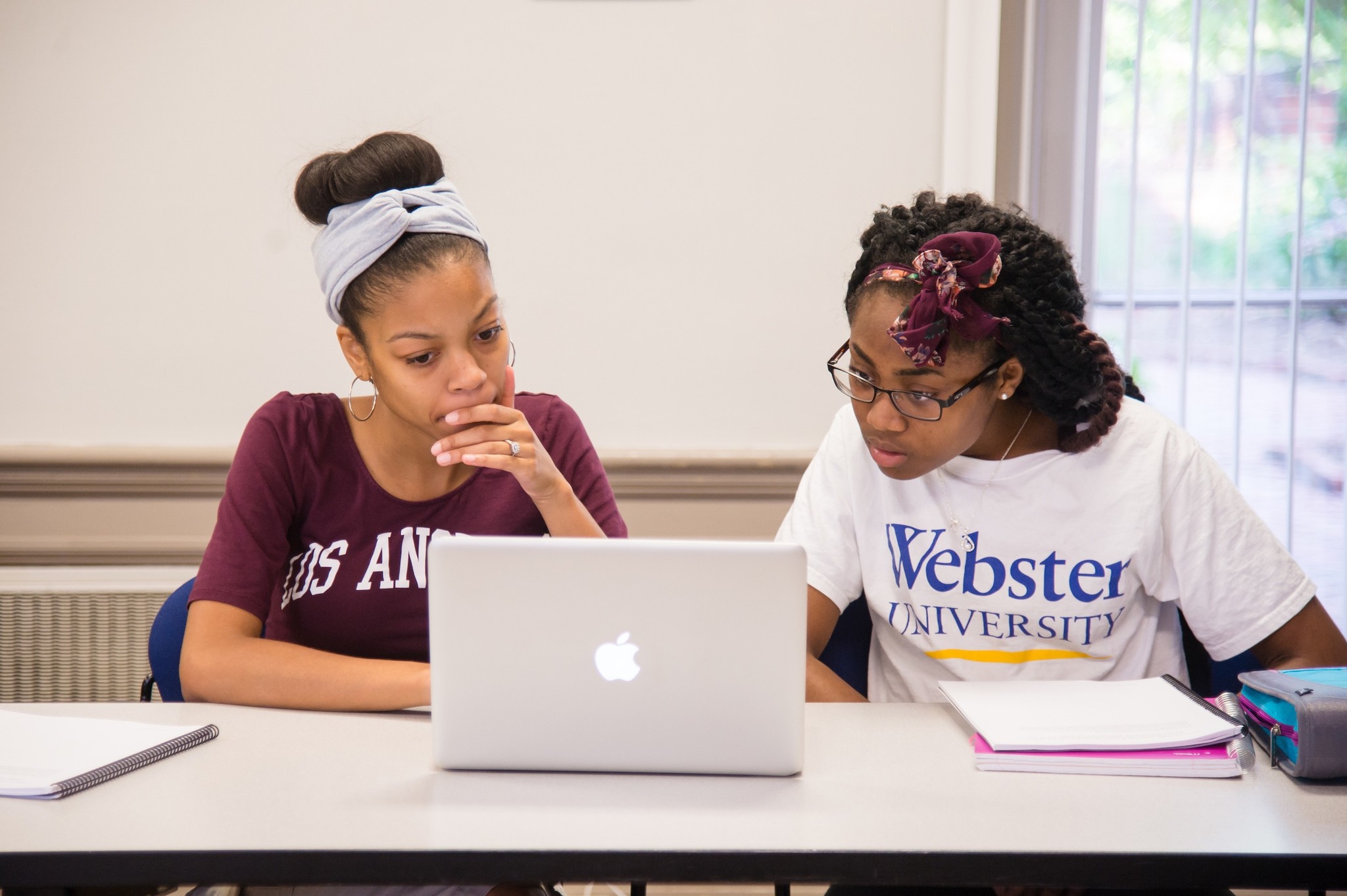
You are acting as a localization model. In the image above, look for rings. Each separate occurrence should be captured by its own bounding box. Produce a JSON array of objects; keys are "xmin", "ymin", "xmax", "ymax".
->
[{"xmin": 506, "ymin": 439, "xmax": 519, "ymax": 456}]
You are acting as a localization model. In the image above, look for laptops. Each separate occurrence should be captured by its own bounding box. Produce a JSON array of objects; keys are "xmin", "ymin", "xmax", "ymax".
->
[{"xmin": 427, "ymin": 534, "xmax": 807, "ymax": 777}]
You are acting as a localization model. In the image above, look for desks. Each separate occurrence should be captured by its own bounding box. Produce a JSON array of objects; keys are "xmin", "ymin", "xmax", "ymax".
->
[{"xmin": 0, "ymin": 703, "xmax": 1347, "ymax": 896}]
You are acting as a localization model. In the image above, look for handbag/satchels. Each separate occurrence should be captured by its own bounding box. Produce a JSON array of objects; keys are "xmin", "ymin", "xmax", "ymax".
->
[{"xmin": 1235, "ymin": 667, "xmax": 1347, "ymax": 780}]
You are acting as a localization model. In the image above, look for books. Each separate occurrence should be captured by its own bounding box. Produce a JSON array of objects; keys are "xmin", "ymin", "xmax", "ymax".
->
[
  {"xmin": 971, "ymin": 690, "xmax": 1256, "ymax": 778},
  {"xmin": 932, "ymin": 673, "xmax": 1249, "ymax": 752},
  {"xmin": 0, "ymin": 709, "xmax": 219, "ymax": 799}
]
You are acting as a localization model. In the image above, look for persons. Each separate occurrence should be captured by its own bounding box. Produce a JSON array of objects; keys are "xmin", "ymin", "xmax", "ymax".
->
[
  {"xmin": 774, "ymin": 190, "xmax": 1347, "ymax": 896},
  {"xmin": 181, "ymin": 131, "xmax": 628, "ymax": 896}
]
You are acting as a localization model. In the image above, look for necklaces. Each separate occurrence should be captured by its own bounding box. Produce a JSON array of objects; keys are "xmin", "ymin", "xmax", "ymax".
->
[{"xmin": 939, "ymin": 406, "xmax": 1033, "ymax": 552}]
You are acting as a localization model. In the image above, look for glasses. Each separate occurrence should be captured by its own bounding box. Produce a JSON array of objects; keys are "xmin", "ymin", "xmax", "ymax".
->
[{"xmin": 827, "ymin": 339, "xmax": 1012, "ymax": 421}]
